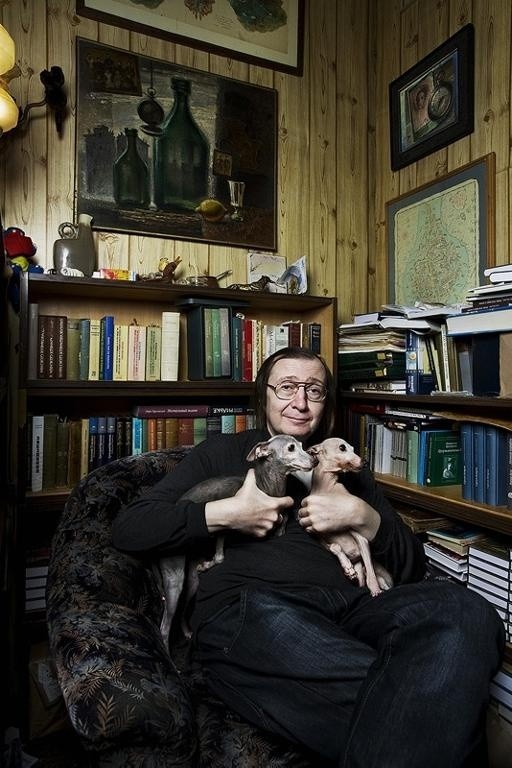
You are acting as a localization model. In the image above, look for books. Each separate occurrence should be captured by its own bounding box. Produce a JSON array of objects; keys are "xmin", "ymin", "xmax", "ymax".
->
[
  {"xmin": 28, "ymin": 303, "xmax": 321, "ymax": 383},
  {"xmin": 396, "ymin": 512, "xmax": 512, "ymax": 742},
  {"xmin": 20, "ymin": 549, "xmax": 46, "ymax": 610},
  {"xmin": 335, "ymin": 263, "xmax": 512, "ymax": 508},
  {"xmin": 28, "ymin": 402, "xmax": 258, "ymax": 492}
]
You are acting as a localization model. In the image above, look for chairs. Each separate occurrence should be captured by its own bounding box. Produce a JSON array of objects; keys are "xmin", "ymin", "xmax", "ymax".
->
[{"xmin": 45, "ymin": 445, "xmax": 338, "ymax": 768}]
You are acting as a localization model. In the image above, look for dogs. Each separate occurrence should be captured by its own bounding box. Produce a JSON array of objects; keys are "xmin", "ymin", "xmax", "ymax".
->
[
  {"xmin": 305, "ymin": 437, "xmax": 394, "ymax": 598},
  {"xmin": 153, "ymin": 434, "xmax": 320, "ymax": 674}
]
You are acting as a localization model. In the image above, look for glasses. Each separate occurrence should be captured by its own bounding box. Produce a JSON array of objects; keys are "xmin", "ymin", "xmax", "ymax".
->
[{"xmin": 263, "ymin": 380, "xmax": 331, "ymax": 403}]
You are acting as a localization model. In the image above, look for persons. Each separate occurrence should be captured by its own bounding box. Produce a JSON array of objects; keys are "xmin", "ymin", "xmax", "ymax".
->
[{"xmin": 114, "ymin": 344, "xmax": 506, "ymax": 768}]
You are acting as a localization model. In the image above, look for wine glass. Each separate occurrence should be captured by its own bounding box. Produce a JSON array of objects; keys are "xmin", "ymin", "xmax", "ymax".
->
[{"xmin": 226, "ymin": 178, "xmax": 247, "ymax": 222}]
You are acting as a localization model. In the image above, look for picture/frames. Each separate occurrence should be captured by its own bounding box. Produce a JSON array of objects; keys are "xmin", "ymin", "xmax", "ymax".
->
[
  {"xmin": 75, "ymin": 0, "xmax": 305, "ymax": 77},
  {"xmin": 74, "ymin": 35, "xmax": 279, "ymax": 255},
  {"xmin": 388, "ymin": 22, "xmax": 476, "ymax": 173},
  {"xmin": 383, "ymin": 151, "xmax": 496, "ymax": 308}
]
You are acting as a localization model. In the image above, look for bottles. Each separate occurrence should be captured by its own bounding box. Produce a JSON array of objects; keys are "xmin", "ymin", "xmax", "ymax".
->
[
  {"xmin": 155, "ymin": 76, "xmax": 210, "ymax": 211},
  {"xmin": 110, "ymin": 126, "xmax": 151, "ymax": 206}
]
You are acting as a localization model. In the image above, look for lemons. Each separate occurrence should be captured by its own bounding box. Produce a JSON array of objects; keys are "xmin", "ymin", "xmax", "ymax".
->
[{"xmin": 195, "ymin": 199, "xmax": 227, "ymax": 222}]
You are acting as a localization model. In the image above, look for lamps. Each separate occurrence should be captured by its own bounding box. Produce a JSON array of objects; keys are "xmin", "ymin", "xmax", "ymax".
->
[{"xmin": 0, "ymin": 20, "xmax": 67, "ymax": 154}]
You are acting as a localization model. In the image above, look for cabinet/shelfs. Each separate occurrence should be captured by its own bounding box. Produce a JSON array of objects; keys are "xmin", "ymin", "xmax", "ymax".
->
[
  {"xmin": 12, "ymin": 269, "xmax": 339, "ymax": 744},
  {"xmin": 340, "ymin": 384, "xmax": 512, "ymax": 540}
]
